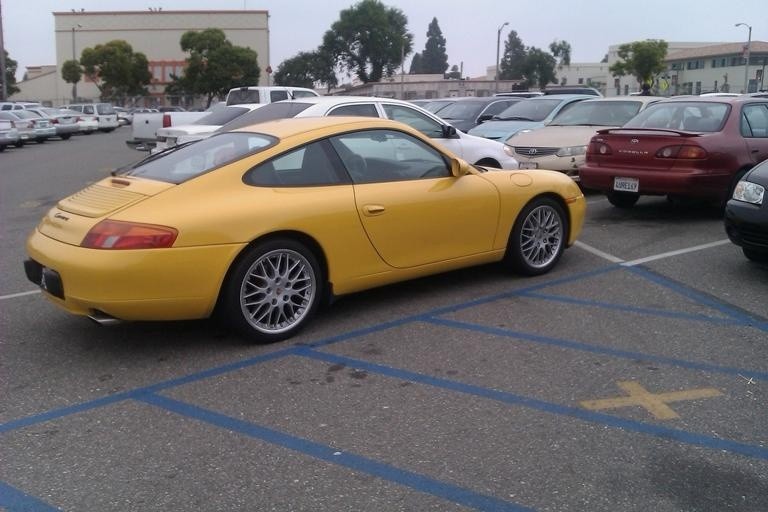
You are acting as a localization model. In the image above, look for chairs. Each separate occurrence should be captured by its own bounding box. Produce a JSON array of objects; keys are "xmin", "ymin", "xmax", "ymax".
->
[
  {"xmin": 645, "ymin": 114, "xmax": 752, "ymax": 137},
  {"xmin": 213, "ymin": 140, "xmax": 365, "ymax": 186}
]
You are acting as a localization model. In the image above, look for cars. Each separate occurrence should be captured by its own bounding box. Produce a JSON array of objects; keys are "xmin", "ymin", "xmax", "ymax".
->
[
  {"xmin": 0, "ymin": 96, "xmax": 226, "ymax": 154},
  {"xmin": 574, "ymin": 96, "xmax": 768, "ymax": 213},
  {"xmin": 148, "ymin": 82, "xmax": 767, "ymax": 192},
  {"xmin": 723, "ymin": 158, "xmax": 768, "ymax": 268}
]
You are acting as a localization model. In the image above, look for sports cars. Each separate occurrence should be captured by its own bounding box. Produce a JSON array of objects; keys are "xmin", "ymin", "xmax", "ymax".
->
[{"xmin": 19, "ymin": 110, "xmax": 594, "ymax": 346}]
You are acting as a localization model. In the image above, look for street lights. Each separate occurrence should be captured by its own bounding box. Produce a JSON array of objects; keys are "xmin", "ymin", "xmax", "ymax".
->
[
  {"xmin": 71, "ymin": 24, "xmax": 85, "ymax": 102},
  {"xmin": 399, "ymin": 34, "xmax": 408, "ymax": 100},
  {"xmin": 494, "ymin": 19, "xmax": 509, "ymax": 92},
  {"xmin": 733, "ymin": 21, "xmax": 752, "ymax": 93},
  {"xmin": 264, "ymin": 65, "xmax": 273, "ymax": 86}
]
